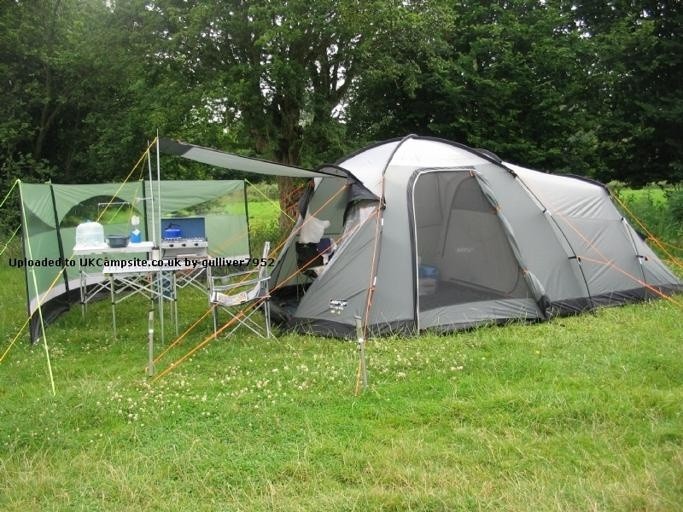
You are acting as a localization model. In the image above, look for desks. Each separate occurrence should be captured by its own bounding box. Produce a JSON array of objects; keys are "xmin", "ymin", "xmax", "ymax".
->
[
  {"xmin": 72, "ymin": 240, "xmax": 153, "ymax": 322},
  {"xmin": 103, "ymin": 260, "xmax": 208, "ymax": 341}
]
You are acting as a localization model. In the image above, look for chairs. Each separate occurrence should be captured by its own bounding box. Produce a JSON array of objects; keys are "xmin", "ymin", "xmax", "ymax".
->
[
  {"xmin": 295, "ymin": 238, "xmax": 338, "ymax": 303},
  {"xmin": 209, "ymin": 242, "xmax": 273, "ymax": 342}
]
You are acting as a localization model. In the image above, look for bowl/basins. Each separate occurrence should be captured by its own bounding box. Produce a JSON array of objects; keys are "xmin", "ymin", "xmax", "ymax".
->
[{"xmin": 105, "ymin": 234, "xmax": 130, "ymax": 248}]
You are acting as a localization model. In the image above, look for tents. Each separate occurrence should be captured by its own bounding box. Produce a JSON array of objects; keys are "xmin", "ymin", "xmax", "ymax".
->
[{"xmin": 163, "ymin": 134, "xmax": 683, "ymax": 340}]
